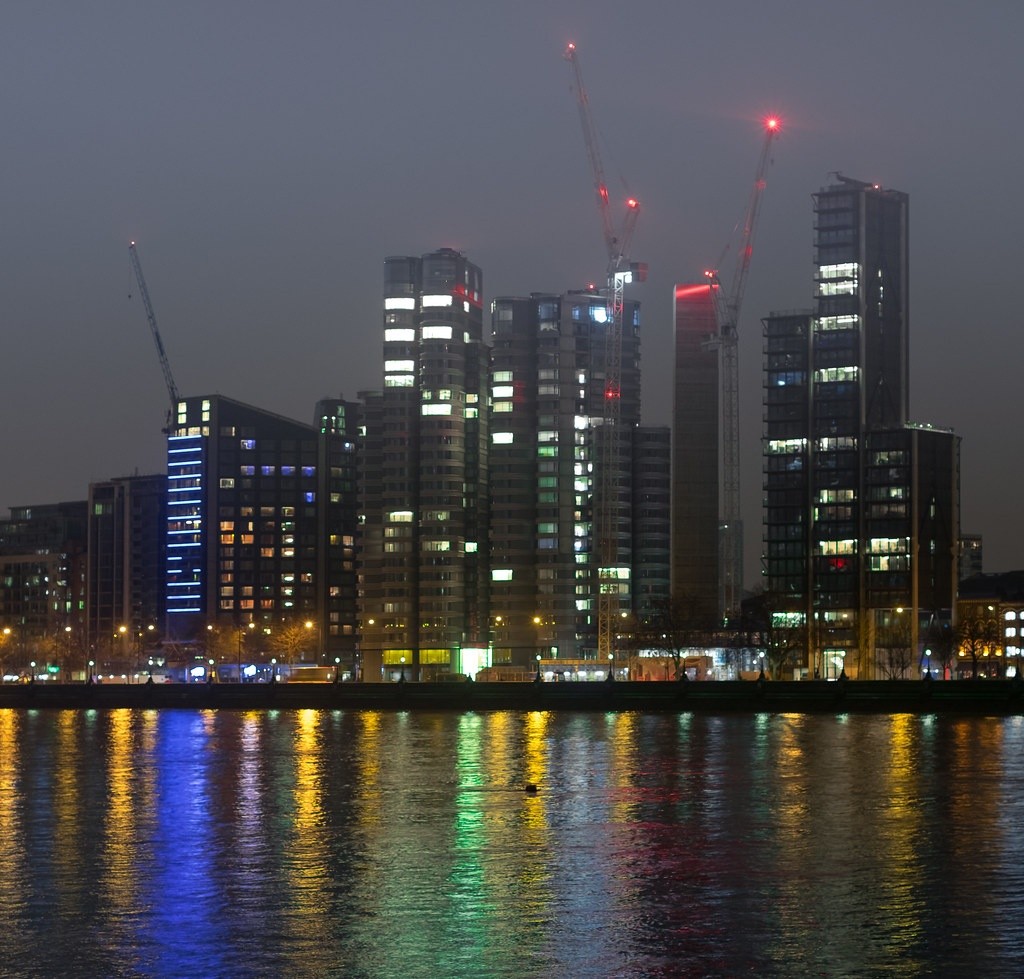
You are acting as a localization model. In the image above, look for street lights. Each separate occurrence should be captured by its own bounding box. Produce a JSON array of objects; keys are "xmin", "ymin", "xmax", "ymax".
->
[
  {"xmin": 271, "ymin": 657, "xmax": 277, "ymax": 683},
  {"xmin": 603, "ymin": 654, "xmax": 616, "ymax": 681},
  {"xmin": 334, "ymin": 656, "xmax": 342, "ymax": 684},
  {"xmin": 534, "ymin": 617, "xmax": 549, "ymax": 658},
  {"xmin": 486, "ymin": 615, "xmax": 502, "ymax": 682},
  {"xmin": 145, "ymin": 659, "xmax": 156, "ymax": 685},
  {"xmin": 358, "ymin": 620, "xmax": 374, "ymax": 665},
  {"xmin": 119, "ymin": 623, "xmax": 140, "ymax": 672},
  {"xmin": 921, "ymin": 648, "xmax": 935, "ymax": 680},
  {"xmin": 238, "ymin": 623, "xmax": 255, "ymax": 683},
  {"xmin": 396, "ymin": 656, "xmax": 409, "ymax": 683},
  {"xmin": 65, "ymin": 626, "xmax": 72, "ymax": 685},
  {"xmin": 31, "ymin": 661, "xmax": 37, "ymax": 685},
  {"xmin": 87, "ymin": 661, "xmax": 96, "ymax": 685},
  {"xmin": 205, "ymin": 659, "xmax": 217, "ymax": 684},
  {"xmin": 535, "ymin": 654, "xmax": 545, "ymax": 682},
  {"xmin": 986, "ymin": 602, "xmax": 1006, "ymax": 677},
  {"xmin": 305, "ymin": 621, "xmax": 322, "ymax": 667}
]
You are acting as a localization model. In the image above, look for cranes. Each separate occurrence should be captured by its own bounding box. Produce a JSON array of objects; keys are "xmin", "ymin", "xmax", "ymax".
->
[
  {"xmin": 564, "ymin": 39, "xmax": 643, "ymax": 655},
  {"xmin": 126, "ymin": 238, "xmax": 182, "ymax": 432},
  {"xmin": 710, "ymin": 115, "xmax": 780, "ymax": 622}
]
